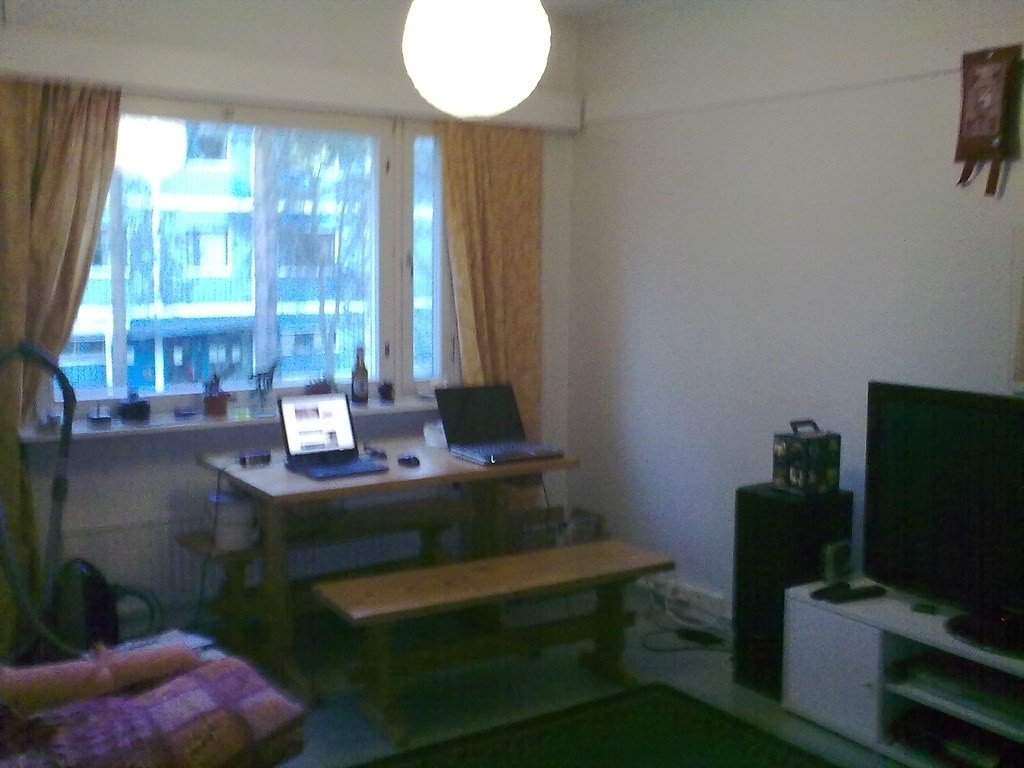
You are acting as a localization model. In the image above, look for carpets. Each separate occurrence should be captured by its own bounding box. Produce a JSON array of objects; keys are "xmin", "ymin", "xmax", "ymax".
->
[{"xmin": 367, "ymin": 682, "xmax": 838, "ymax": 768}]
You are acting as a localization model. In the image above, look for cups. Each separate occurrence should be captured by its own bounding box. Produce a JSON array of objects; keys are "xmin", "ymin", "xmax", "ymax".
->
[{"xmin": 824, "ymin": 540, "xmax": 851, "ymax": 585}]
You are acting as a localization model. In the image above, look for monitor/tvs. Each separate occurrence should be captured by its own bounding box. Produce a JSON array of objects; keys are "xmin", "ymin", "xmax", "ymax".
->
[{"xmin": 862, "ymin": 380, "xmax": 1024, "ymax": 653}]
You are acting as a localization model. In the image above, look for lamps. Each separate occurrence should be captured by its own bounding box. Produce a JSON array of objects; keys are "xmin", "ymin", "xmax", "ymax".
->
[{"xmin": 402, "ymin": 1, "xmax": 552, "ymax": 121}]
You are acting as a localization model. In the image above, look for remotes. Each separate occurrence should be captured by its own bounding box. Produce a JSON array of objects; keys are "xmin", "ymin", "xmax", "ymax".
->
[{"xmin": 811, "ymin": 580, "xmax": 886, "ymax": 604}]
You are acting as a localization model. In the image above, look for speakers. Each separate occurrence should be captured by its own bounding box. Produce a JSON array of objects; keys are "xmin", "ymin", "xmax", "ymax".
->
[{"xmin": 731, "ymin": 483, "xmax": 855, "ymax": 701}]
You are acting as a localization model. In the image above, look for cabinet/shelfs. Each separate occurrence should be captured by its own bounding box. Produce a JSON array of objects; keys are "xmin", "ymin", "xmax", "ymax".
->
[{"xmin": 782, "ymin": 577, "xmax": 1024, "ymax": 768}]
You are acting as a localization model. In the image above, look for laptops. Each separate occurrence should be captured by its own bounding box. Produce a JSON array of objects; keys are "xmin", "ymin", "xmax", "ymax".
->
[
  {"xmin": 434, "ymin": 383, "xmax": 564, "ymax": 466},
  {"xmin": 277, "ymin": 393, "xmax": 389, "ymax": 480}
]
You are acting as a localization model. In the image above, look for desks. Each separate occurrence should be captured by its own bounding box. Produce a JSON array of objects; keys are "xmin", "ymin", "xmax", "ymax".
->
[{"xmin": 195, "ymin": 433, "xmax": 583, "ymax": 709}]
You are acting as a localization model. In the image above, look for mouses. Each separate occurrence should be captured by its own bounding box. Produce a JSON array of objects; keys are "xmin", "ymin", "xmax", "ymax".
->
[{"xmin": 398, "ymin": 456, "xmax": 420, "ymax": 467}]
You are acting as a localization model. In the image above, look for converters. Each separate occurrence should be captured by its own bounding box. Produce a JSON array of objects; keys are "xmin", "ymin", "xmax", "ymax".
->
[{"xmin": 240, "ymin": 452, "xmax": 271, "ymax": 465}]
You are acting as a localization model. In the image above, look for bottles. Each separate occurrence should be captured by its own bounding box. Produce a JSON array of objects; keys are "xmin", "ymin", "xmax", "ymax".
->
[{"xmin": 351, "ymin": 348, "xmax": 368, "ymax": 402}]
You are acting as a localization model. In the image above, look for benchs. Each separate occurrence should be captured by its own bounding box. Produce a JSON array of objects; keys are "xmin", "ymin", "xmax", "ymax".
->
[
  {"xmin": 311, "ymin": 541, "xmax": 673, "ymax": 746},
  {"xmin": 177, "ymin": 491, "xmax": 550, "ymax": 654}
]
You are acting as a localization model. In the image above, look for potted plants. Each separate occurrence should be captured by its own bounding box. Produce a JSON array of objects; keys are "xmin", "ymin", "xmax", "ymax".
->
[
  {"xmin": 202, "ymin": 371, "xmax": 230, "ymax": 414},
  {"xmin": 306, "ymin": 373, "xmax": 335, "ymax": 394}
]
